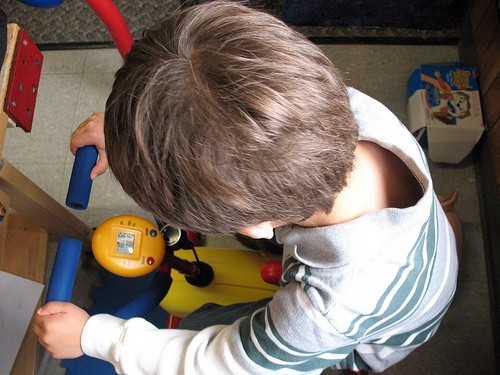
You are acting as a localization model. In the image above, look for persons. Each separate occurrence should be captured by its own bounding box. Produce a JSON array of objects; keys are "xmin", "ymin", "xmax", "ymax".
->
[{"xmin": 32, "ymin": 0, "xmax": 459, "ymax": 375}]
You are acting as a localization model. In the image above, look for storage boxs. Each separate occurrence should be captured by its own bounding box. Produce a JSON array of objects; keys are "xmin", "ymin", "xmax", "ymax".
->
[{"xmin": 407, "ymin": 59, "xmax": 485, "ymax": 165}]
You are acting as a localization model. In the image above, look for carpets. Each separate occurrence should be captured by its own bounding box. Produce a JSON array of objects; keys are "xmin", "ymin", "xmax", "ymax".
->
[{"xmin": 0, "ymin": 0, "xmax": 460, "ymax": 52}]
